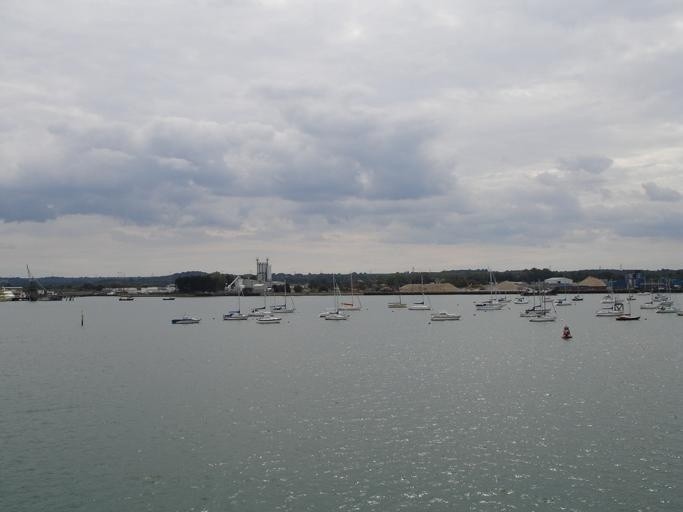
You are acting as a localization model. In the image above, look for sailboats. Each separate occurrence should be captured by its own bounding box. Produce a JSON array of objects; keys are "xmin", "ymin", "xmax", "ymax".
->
[
  {"xmin": 385, "ymin": 273, "xmax": 432, "ymax": 310},
  {"xmin": 594, "ymin": 277, "xmax": 683, "ymax": 322},
  {"xmin": 474, "ymin": 269, "xmax": 571, "ymax": 323},
  {"xmin": 222, "ymin": 279, "xmax": 298, "ymax": 325},
  {"xmin": 318, "ymin": 271, "xmax": 364, "ymax": 320}
]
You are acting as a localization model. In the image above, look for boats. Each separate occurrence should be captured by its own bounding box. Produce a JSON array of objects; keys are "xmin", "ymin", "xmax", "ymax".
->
[
  {"xmin": 561, "ymin": 327, "xmax": 571, "ymax": 340},
  {"xmin": 428, "ymin": 311, "xmax": 462, "ymax": 321},
  {"xmin": 118, "ymin": 298, "xmax": 133, "ymax": 301},
  {"xmin": 162, "ymin": 297, "xmax": 174, "ymax": 300},
  {"xmin": 573, "ymin": 293, "xmax": 583, "ymax": 302},
  {"xmin": 171, "ymin": 312, "xmax": 199, "ymax": 324}
]
